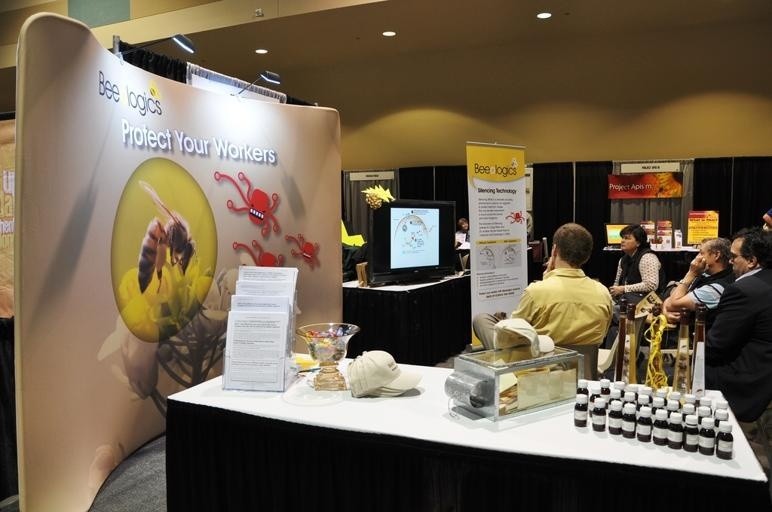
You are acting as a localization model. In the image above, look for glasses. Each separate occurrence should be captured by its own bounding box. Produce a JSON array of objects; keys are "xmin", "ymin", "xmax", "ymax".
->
[{"xmin": 729, "ymin": 252, "xmax": 745, "ymax": 259}]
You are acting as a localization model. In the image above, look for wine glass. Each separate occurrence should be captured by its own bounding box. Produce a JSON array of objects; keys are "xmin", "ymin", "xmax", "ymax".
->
[{"xmin": 295, "ymin": 323, "xmax": 360, "ymax": 391}]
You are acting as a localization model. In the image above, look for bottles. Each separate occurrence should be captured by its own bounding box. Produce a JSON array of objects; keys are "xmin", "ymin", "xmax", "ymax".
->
[{"xmin": 574, "ymin": 297, "xmax": 734, "ymax": 460}]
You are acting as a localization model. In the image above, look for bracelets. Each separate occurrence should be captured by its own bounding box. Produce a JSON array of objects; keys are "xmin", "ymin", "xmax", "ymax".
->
[{"xmin": 679, "ymin": 279, "xmax": 689, "ymax": 287}]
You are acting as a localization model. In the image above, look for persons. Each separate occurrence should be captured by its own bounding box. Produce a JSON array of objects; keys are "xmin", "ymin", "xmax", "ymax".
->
[
  {"xmin": 639, "ymin": 225, "xmax": 772, "ymax": 424},
  {"xmin": 471, "ymin": 221, "xmax": 615, "ymax": 350},
  {"xmin": 597, "ymin": 223, "xmax": 667, "ymax": 349},
  {"xmin": 455, "ymin": 218, "xmax": 472, "ymax": 246},
  {"xmin": 661, "ymin": 237, "xmax": 736, "ymax": 325}
]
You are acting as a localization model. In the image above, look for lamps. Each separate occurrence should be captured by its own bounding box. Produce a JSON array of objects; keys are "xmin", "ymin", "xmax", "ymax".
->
[
  {"xmin": 230, "ymin": 70, "xmax": 281, "ymax": 100},
  {"xmin": 114, "ymin": 31, "xmax": 196, "ymax": 66}
]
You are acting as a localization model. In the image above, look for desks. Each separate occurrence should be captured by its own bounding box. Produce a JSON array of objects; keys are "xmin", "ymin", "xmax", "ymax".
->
[{"xmin": 144, "ymin": 348, "xmax": 771, "ymax": 505}]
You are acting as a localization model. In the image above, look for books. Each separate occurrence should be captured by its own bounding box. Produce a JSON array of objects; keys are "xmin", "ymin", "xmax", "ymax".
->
[{"xmin": 223, "ymin": 264, "xmax": 300, "ymax": 392}]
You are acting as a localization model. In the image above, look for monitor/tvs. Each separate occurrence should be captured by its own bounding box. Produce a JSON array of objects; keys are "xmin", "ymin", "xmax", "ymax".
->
[{"xmin": 368, "ymin": 198, "xmax": 456, "ymax": 283}]
